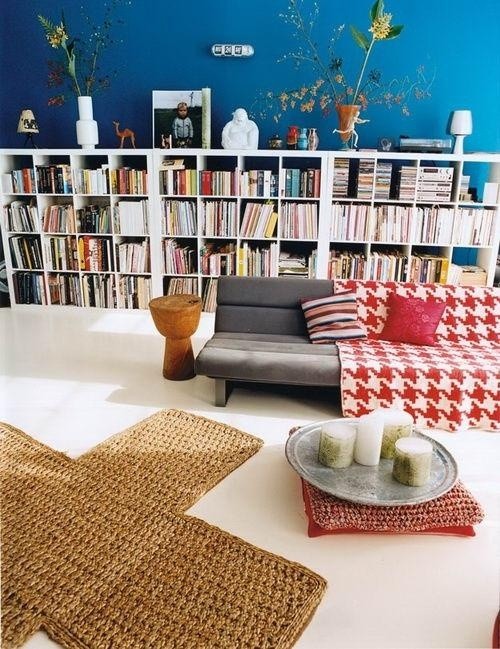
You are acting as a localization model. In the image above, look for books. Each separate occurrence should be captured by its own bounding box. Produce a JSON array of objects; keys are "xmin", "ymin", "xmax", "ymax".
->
[
  {"xmin": 451, "ymin": 179, "xmax": 497, "ymax": 286},
  {"xmin": 111, "ymin": 166, "xmax": 152, "ymax": 309},
  {"xmin": 73, "ymin": 165, "xmax": 117, "ymax": 308},
  {"xmin": 370, "ymin": 164, "xmax": 417, "ymax": 282},
  {"xmin": 2, "ymin": 169, "xmax": 48, "ymax": 304},
  {"xmin": 329, "ymin": 160, "xmax": 375, "ymax": 280},
  {"xmin": 278, "ymin": 168, "xmax": 320, "ymax": 279},
  {"xmin": 239, "ymin": 171, "xmax": 278, "ymax": 277},
  {"xmin": 199, "ymin": 171, "xmax": 237, "ymax": 312},
  {"xmin": 410, "ymin": 168, "xmax": 453, "ymax": 283},
  {"xmin": 35, "ymin": 164, "xmax": 82, "ymax": 307},
  {"xmin": 159, "ymin": 159, "xmax": 199, "ymax": 296}
]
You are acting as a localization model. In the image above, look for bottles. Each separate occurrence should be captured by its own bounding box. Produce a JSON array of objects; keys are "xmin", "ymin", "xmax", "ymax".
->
[
  {"xmin": 319, "ymin": 408, "xmax": 434, "ymax": 487},
  {"xmin": 268, "ymin": 126, "xmax": 319, "ymax": 150}
]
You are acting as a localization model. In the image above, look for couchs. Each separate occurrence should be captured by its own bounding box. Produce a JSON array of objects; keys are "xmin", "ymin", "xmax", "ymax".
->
[{"xmin": 194, "ymin": 274, "xmax": 500, "ymax": 408}]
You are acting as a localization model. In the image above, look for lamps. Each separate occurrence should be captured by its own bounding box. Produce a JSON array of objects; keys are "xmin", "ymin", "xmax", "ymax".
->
[
  {"xmin": 14, "ymin": 108, "xmax": 40, "ymax": 148},
  {"xmin": 449, "ymin": 109, "xmax": 474, "ymax": 157}
]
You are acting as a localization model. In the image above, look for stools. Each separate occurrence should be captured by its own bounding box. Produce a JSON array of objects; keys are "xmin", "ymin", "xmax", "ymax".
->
[{"xmin": 150, "ymin": 294, "xmax": 203, "ymax": 381}]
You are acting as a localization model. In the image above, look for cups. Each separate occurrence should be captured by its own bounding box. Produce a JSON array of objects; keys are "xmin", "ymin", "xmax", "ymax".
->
[{"xmin": 482, "ymin": 182, "xmax": 499, "ymax": 206}]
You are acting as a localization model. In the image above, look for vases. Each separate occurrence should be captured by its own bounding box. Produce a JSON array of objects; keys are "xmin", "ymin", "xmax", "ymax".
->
[
  {"xmin": 333, "ymin": 104, "xmax": 370, "ymax": 149},
  {"xmin": 286, "ymin": 126, "xmax": 320, "ymax": 150},
  {"xmin": 75, "ymin": 94, "xmax": 99, "ymax": 149}
]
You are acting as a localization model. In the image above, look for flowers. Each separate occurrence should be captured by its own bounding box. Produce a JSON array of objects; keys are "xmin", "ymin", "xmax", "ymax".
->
[
  {"xmin": 35, "ymin": 0, "xmax": 133, "ymax": 106},
  {"xmin": 230, "ymin": 0, "xmax": 438, "ymax": 126}
]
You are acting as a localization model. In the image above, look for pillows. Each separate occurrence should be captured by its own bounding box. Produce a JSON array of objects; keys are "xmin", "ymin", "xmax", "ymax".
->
[
  {"xmin": 297, "ymin": 291, "xmax": 368, "ymax": 346},
  {"xmin": 379, "ymin": 293, "xmax": 447, "ymax": 350}
]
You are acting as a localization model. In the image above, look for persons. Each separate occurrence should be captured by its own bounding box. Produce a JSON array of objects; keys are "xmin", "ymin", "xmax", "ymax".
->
[
  {"xmin": 172, "ymin": 103, "xmax": 194, "ymax": 148},
  {"xmin": 220, "ymin": 108, "xmax": 259, "ymax": 150}
]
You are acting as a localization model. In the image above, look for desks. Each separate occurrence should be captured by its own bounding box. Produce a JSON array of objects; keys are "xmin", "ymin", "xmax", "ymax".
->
[{"xmin": 0, "ymin": 402, "xmax": 500, "ymax": 649}]
